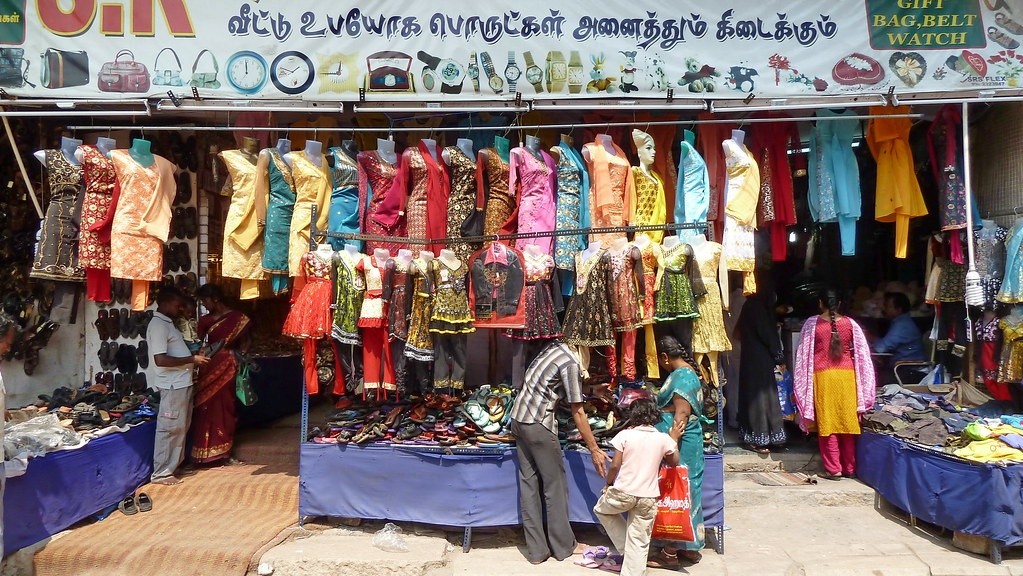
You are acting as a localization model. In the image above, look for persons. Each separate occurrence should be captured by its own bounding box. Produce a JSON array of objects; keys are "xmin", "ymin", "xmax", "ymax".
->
[
  {"xmin": 869, "ymin": 292, "xmax": 928, "ymax": 386},
  {"xmin": 732, "ymin": 285, "xmax": 788, "ymax": 453},
  {"xmin": 284, "ymin": 235, "xmax": 732, "ymax": 394},
  {"xmin": 510, "ymin": 341, "xmax": 613, "ymax": 564},
  {"xmin": 220, "ymin": 128, "xmax": 761, "ymax": 280},
  {"xmin": 145, "ymin": 288, "xmax": 210, "ymax": 484},
  {"xmin": 792, "ymin": 288, "xmax": 876, "ymax": 481},
  {"xmin": 30, "ymin": 138, "xmax": 176, "ymax": 302},
  {"xmin": 189, "ymin": 284, "xmax": 249, "ymax": 463},
  {"xmin": 935, "ymin": 215, "xmax": 1023, "ymax": 383},
  {"xmin": 645, "ymin": 336, "xmax": 706, "ymax": 570},
  {"xmin": 593, "ymin": 398, "xmax": 685, "ymax": 576}
]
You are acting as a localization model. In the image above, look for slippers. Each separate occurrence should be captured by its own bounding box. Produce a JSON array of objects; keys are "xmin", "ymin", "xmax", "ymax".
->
[
  {"xmin": 746, "ymin": 443, "xmax": 772, "ymax": 454},
  {"xmin": 306, "ymin": 366, "xmax": 726, "ymax": 456},
  {"xmin": 842, "ymin": 472, "xmax": 857, "ymax": 479},
  {"xmin": 195, "ymin": 338, "xmax": 225, "ymax": 370},
  {"xmin": 816, "ymin": 470, "xmax": 842, "ymax": 481},
  {"xmin": 573, "ymin": 558, "xmax": 604, "ymax": 568},
  {"xmin": 598, "ymin": 559, "xmax": 622, "ymax": 572},
  {"xmin": 583, "ymin": 548, "xmax": 595, "ymax": 562},
  {"xmin": 606, "ymin": 554, "xmax": 624, "ymax": 564},
  {"xmin": 596, "ymin": 545, "xmax": 609, "ymax": 559},
  {"xmin": 675, "ymin": 547, "xmax": 702, "ymax": 564},
  {"xmin": 0, "ymin": 112, "xmax": 198, "ymax": 435},
  {"xmin": 646, "ymin": 551, "xmax": 682, "ymax": 571},
  {"xmin": 116, "ymin": 491, "xmax": 153, "ymax": 515},
  {"xmin": 771, "ymin": 443, "xmax": 786, "ymax": 453}
]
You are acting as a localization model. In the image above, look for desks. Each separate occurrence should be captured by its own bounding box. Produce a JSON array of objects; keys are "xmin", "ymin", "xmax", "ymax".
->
[
  {"xmin": 848, "ymin": 399, "xmax": 1023, "ymax": 563},
  {"xmin": 1, "ymin": 405, "xmax": 163, "ymax": 559},
  {"xmin": 298, "ymin": 438, "xmax": 725, "ymax": 557}
]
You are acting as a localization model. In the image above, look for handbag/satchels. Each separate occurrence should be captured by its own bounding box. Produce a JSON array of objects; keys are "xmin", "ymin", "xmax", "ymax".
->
[
  {"xmin": 0, "ymin": 48, "xmax": 221, "ymax": 93},
  {"xmin": 234, "ymin": 355, "xmax": 258, "ymax": 407},
  {"xmin": 774, "ymin": 361, "xmax": 798, "ymax": 421},
  {"xmin": 648, "ymin": 461, "xmax": 697, "ymax": 544}
]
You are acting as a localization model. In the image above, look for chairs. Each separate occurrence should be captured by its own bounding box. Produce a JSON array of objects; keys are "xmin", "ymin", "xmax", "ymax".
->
[{"xmin": 890, "ymin": 328, "xmax": 936, "ymax": 386}]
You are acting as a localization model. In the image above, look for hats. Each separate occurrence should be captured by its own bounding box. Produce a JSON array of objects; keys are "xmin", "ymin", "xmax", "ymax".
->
[
  {"xmin": 884, "ymin": 279, "xmax": 909, "ymax": 294},
  {"xmin": 850, "ymin": 285, "xmax": 871, "ymax": 301}
]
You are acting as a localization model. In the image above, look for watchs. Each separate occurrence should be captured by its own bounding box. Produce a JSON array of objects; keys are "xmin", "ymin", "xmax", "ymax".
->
[{"xmin": 418, "ymin": 51, "xmax": 583, "ymax": 93}]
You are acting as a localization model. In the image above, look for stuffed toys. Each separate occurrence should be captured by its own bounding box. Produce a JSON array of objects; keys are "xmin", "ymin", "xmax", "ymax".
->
[
  {"xmin": 677, "ymin": 57, "xmax": 720, "ymax": 93},
  {"xmin": 586, "ymin": 51, "xmax": 617, "ymax": 94},
  {"xmin": 618, "ymin": 51, "xmax": 639, "ymax": 93},
  {"xmin": 724, "ymin": 56, "xmax": 759, "ymax": 92}
]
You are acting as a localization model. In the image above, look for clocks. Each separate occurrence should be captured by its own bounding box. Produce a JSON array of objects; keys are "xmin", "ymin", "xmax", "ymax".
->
[{"xmin": 226, "ymin": 51, "xmax": 361, "ymax": 95}]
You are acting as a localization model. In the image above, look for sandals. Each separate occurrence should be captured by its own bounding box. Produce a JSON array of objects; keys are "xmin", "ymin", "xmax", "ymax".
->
[
  {"xmin": 223, "ymin": 457, "xmax": 246, "ymax": 466},
  {"xmin": 986, "ymin": 26, "xmax": 1020, "ymax": 49},
  {"xmin": 994, "ymin": 12, "xmax": 1023, "ymax": 35}
]
